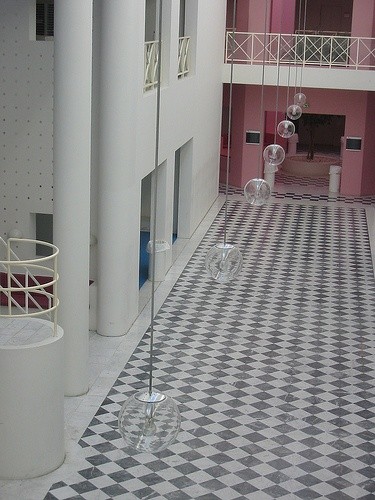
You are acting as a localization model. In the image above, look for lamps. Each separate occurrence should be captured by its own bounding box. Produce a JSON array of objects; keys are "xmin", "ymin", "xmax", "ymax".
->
[{"xmin": 115, "ymin": 0, "xmax": 309, "ymax": 453}]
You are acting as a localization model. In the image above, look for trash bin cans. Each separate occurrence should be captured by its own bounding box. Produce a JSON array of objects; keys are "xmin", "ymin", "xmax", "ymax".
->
[
  {"xmin": 328, "ymin": 165, "xmax": 341, "ymax": 193},
  {"xmin": 145, "ymin": 240, "xmax": 170, "ymax": 282}
]
